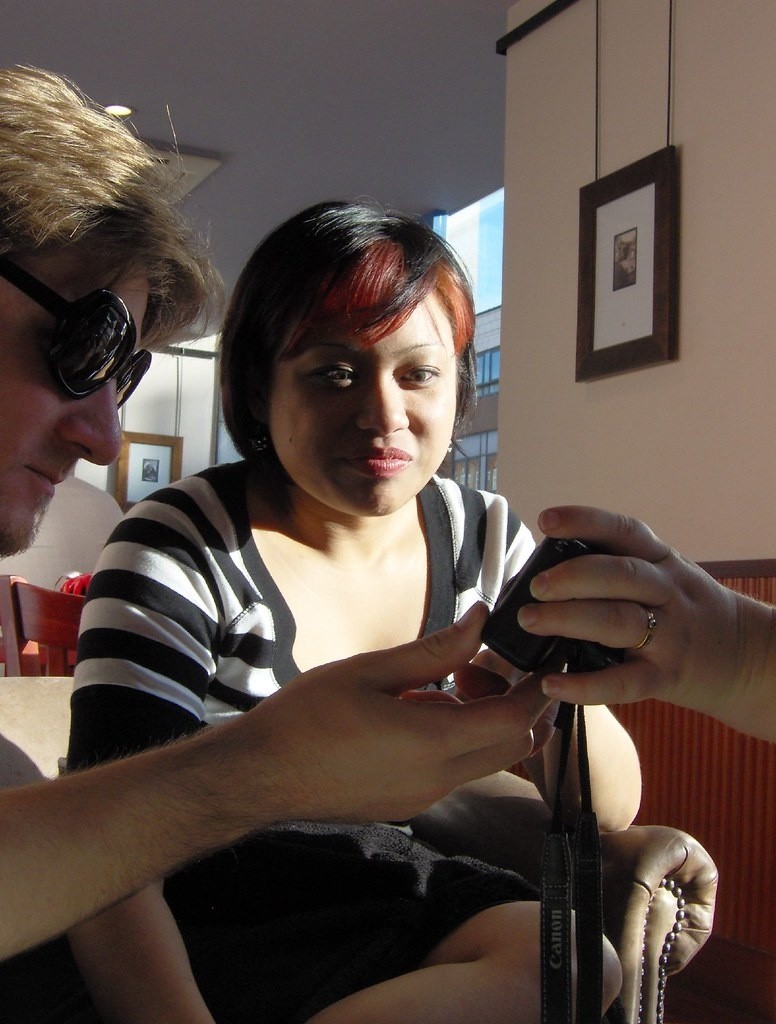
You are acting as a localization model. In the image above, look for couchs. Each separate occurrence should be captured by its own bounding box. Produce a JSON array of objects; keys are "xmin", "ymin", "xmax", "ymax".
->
[{"xmin": 0, "ymin": 669, "xmax": 718, "ymax": 1024}]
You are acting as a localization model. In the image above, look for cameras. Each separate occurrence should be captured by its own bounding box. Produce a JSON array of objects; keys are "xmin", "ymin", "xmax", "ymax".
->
[{"xmin": 476, "ymin": 527, "xmax": 626, "ymax": 681}]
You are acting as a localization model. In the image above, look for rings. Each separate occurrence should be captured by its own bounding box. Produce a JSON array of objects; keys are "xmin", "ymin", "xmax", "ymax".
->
[{"xmin": 634, "ymin": 605, "xmax": 657, "ymax": 650}]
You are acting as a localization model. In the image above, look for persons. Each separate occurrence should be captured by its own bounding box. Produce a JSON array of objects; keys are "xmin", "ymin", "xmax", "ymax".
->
[
  {"xmin": 67, "ymin": 198, "xmax": 642, "ymax": 1024},
  {"xmin": 517, "ymin": 504, "xmax": 776, "ymax": 744},
  {"xmin": 0, "ymin": 65, "xmax": 564, "ymax": 1024}
]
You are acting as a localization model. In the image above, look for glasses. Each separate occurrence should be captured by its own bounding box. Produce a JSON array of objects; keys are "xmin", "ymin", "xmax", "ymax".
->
[{"xmin": 0, "ymin": 257, "xmax": 152, "ymax": 411}]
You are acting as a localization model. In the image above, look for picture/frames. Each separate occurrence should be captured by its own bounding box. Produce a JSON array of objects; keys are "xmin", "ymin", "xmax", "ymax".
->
[
  {"xmin": 116, "ymin": 432, "xmax": 184, "ymax": 514},
  {"xmin": 574, "ymin": 145, "xmax": 678, "ymax": 382}
]
